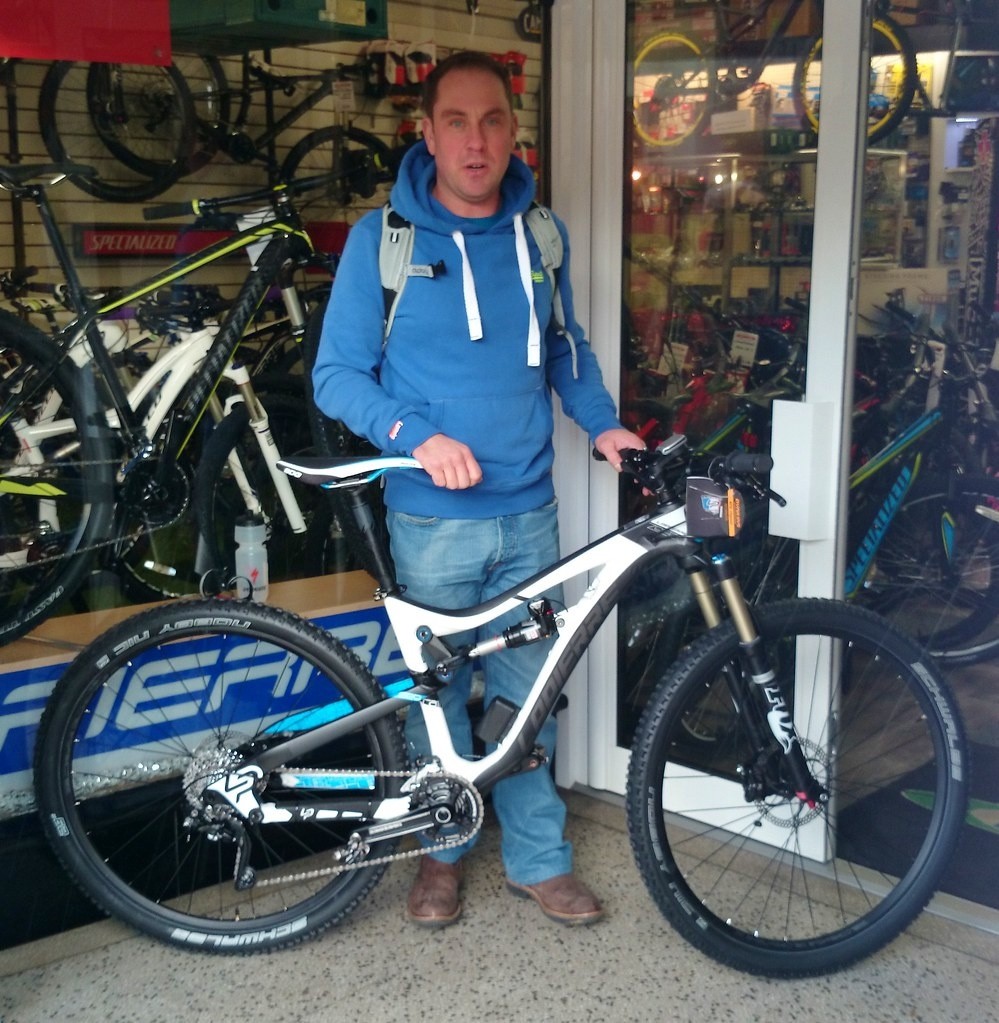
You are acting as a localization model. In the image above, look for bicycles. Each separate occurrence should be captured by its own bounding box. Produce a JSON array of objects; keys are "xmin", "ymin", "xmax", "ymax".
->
[
  {"xmin": 0, "ymin": 0, "xmax": 998, "ymax": 754},
  {"xmin": 33, "ymin": 445, "xmax": 970, "ymax": 981}
]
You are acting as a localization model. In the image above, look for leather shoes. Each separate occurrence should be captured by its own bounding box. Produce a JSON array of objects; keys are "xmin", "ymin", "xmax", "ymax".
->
[
  {"xmin": 405, "ymin": 852, "xmax": 464, "ymax": 930},
  {"xmin": 504, "ymin": 875, "xmax": 604, "ymax": 929}
]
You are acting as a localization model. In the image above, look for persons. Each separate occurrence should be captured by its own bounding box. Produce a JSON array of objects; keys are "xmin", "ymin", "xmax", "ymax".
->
[{"xmin": 315, "ymin": 52, "xmax": 644, "ymax": 928}]
